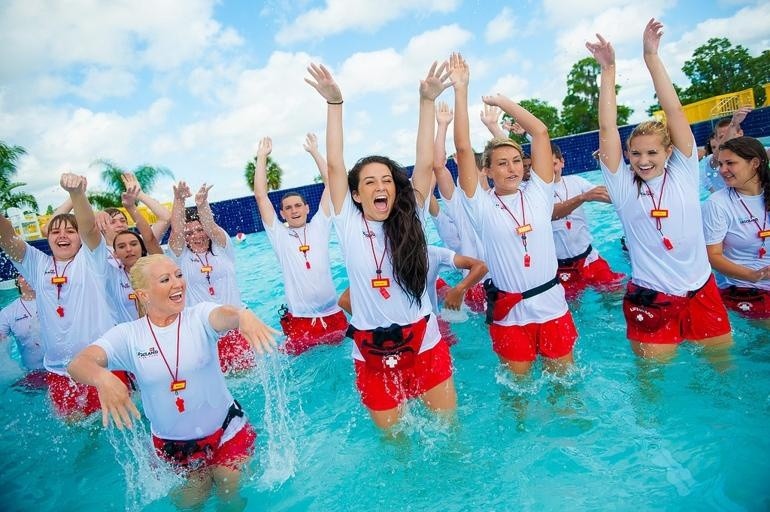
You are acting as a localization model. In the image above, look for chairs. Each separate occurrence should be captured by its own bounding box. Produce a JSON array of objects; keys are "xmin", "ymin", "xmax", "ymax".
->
[{"xmin": 5, "ymin": 205, "xmax": 43, "ymax": 243}]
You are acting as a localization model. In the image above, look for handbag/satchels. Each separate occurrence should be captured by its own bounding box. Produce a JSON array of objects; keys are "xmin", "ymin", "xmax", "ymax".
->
[
  {"xmin": 151, "ymin": 426, "xmax": 225, "ymax": 474},
  {"xmin": 554, "ymin": 256, "xmax": 587, "ymax": 284},
  {"xmin": 622, "ymin": 290, "xmax": 670, "ymax": 333},
  {"xmin": 483, "ymin": 278, "xmax": 522, "ymax": 322},
  {"xmin": 351, "ymin": 317, "xmax": 429, "ymax": 374},
  {"xmin": 718, "ymin": 285, "xmax": 770, "ymax": 322}
]
